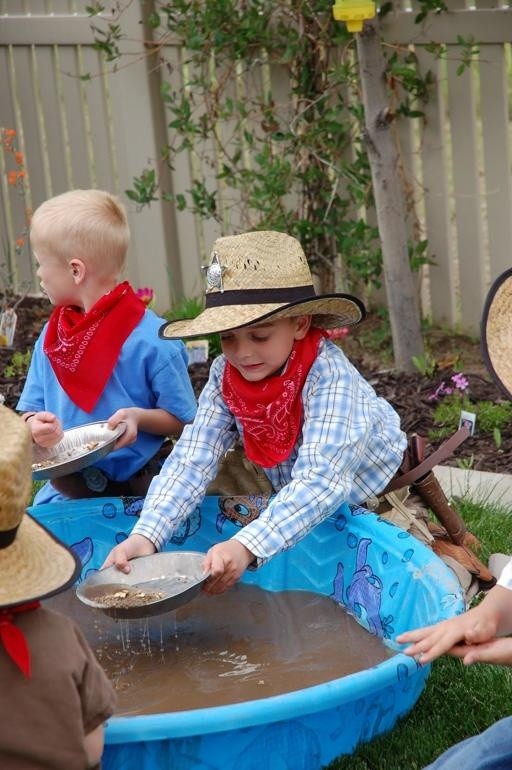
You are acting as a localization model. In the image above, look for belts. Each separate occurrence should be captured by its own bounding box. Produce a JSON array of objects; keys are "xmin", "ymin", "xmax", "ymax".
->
[{"xmin": 354, "ymin": 414, "xmax": 475, "ymax": 517}]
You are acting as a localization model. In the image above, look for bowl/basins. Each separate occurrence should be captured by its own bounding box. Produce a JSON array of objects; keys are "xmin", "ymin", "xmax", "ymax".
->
[{"xmin": 25, "ymin": 492, "xmax": 465, "ymax": 768}]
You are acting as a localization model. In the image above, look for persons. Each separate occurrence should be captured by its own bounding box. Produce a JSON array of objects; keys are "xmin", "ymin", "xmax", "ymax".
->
[
  {"xmin": 13, "ymin": 186, "xmax": 199, "ymax": 505},
  {"xmin": 1, "ymin": 401, "xmax": 120, "ymax": 769},
  {"xmin": 101, "ymin": 229, "xmax": 496, "ymax": 608},
  {"xmin": 396, "ymin": 557, "xmax": 512, "ymax": 770}
]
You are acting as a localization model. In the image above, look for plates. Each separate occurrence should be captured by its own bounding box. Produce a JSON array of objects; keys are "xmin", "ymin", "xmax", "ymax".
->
[{"xmin": 30, "ymin": 420, "xmax": 127, "ymax": 481}]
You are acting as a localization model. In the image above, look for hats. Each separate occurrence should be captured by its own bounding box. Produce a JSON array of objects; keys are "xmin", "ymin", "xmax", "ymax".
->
[
  {"xmin": 476, "ymin": 262, "xmax": 512, "ymax": 405},
  {"xmin": 0, "ymin": 399, "xmax": 85, "ymax": 615},
  {"xmin": 152, "ymin": 225, "xmax": 368, "ymax": 343}
]
